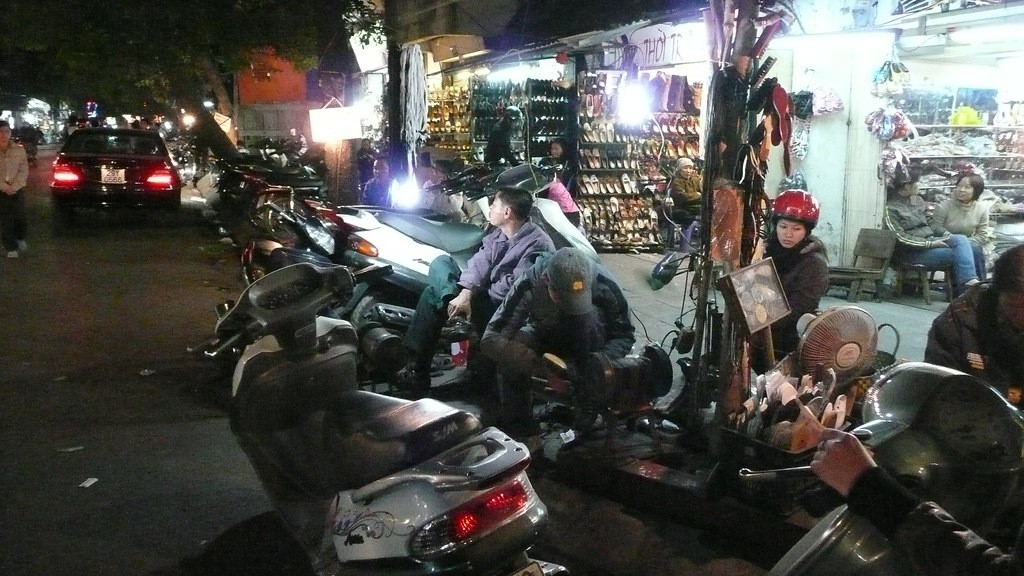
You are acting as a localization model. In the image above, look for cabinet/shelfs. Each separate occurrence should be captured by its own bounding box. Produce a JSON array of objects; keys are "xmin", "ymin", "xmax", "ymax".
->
[
  {"xmin": 903, "ymin": 123, "xmax": 1024, "ymax": 216},
  {"xmin": 425, "ymin": 76, "xmax": 703, "ymax": 254}
]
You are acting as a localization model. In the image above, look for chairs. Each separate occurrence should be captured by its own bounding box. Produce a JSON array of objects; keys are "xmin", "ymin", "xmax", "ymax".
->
[
  {"xmin": 133, "ymin": 138, "xmax": 156, "ymax": 154},
  {"xmin": 826, "ymin": 227, "xmax": 898, "ymax": 303},
  {"xmin": 82, "ymin": 139, "xmax": 107, "ymax": 153},
  {"xmin": 882, "ymin": 216, "xmax": 954, "ymax": 305}
]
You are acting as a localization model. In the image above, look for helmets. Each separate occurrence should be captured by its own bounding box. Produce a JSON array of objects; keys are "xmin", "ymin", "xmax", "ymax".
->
[{"xmin": 771, "ymin": 189, "xmax": 819, "ymax": 228}]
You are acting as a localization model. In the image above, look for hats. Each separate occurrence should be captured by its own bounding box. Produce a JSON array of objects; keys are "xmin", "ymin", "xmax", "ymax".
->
[
  {"xmin": 677, "ymin": 157, "xmax": 693, "ymax": 171},
  {"xmin": 893, "ymin": 172, "xmax": 920, "ymax": 188},
  {"xmin": 549, "ymin": 246, "xmax": 594, "ymax": 314}
]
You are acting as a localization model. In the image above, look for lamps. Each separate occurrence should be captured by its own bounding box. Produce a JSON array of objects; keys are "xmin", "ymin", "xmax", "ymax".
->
[{"xmin": 308, "ymin": 95, "xmax": 363, "ymax": 143}]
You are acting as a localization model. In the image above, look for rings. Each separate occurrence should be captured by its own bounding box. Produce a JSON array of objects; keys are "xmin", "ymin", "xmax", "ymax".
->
[{"xmin": 821, "ymin": 440, "xmax": 827, "ymax": 447}]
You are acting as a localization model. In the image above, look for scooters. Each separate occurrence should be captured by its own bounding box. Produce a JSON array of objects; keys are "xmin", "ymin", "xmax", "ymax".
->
[
  {"xmin": 184, "ymin": 260, "xmax": 570, "ymax": 576},
  {"xmin": 211, "ymin": 129, "xmax": 470, "ymax": 400},
  {"xmin": 738, "ymin": 360, "xmax": 1024, "ymax": 576}
]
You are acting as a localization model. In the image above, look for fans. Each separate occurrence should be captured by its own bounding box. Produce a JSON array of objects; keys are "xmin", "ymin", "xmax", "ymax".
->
[{"xmin": 795, "ymin": 305, "xmax": 880, "ymax": 388}]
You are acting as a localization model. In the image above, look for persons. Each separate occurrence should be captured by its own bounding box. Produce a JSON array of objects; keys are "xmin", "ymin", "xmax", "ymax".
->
[
  {"xmin": 670, "ymin": 157, "xmax": 702, "ymax": 222},
  {"xmin": 195, "ymin": 136, "xmax": 209, "ymax": 172},
  {"xmin": 549, "ymin": 178, "xmax": 581, "ymax": 228},
  {"xmin": 361, "ymin": 157, "xmax": 392, "ymax": 207},
  {"xmin": 0, "ymin": 120, "xmax": 29, "ymax": 258},
  {"xmin": 925, "ymin": 244, "xmax": 1024, "ymax": 410},
  {"xmin": 931, "ymin": 173, "xmax": 989, "ymax": 281},
  {"xmin": 748, "ymin": 190, "xmax": 829, "ymax": 377},
  {"xmin": 882, "ymin": 167, "xmax": 979, "ymax": 291},
  {"xmin": 392, "ymin": 187, "xmax": 556, "ymax": 391},
  {"xmin": 129, "ymin": 118, "xmax": 156, "ymax": 152},
  {"xmin": 549, "ymin": 137, "xmax": 577, "ymax": 199},
  {"xmin": 484, "ymin": 106, "xmax": 524, "ymax": 166},
  {"xmin": 357, "ymin": 139, "xmax": 376, "ymax": 183},
  {"xmin": 480, "ymin": 246, "xmax": 636, "ymax": 437},
  {"xmin": 811, "ymin": 427, "xmax": 1024, "ymax": 576},
  {"xmin": 68, "ymin": 116, "xmax": 98, "ymax": 136}
]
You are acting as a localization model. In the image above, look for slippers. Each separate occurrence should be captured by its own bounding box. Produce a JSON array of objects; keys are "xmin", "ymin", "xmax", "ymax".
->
[
  {"xmin": 427, "ymin": 88, "xmax": 472, "ymax": 152},
  {"xmin": 575, "ymin": 73, "xmax": 700, "ymax": 247}
]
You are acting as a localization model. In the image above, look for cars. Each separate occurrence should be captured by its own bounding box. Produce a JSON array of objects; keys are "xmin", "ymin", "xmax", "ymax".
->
[{"xmin": 48, "ymin": 127, "xmax": 181, "ymax": 231}]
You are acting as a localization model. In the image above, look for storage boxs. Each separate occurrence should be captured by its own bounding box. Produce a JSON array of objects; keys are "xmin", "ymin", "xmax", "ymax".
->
[{"xmin": 718, "ymin": 408, "xmax": 860, "ymax": 508}]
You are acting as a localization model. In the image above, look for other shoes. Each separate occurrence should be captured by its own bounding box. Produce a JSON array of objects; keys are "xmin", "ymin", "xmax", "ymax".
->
[
  {"xmin": 386, "ymin": 368, "xmax": 432, "ymax": 393},
  {"xmin": 6, "ymin": 248, "xmax": 19, "ymax": 258},
  {"xmin": 961, "ymin": 281, "xmax": 985, "ymax": 290},
  {"xmin": 16, "ymin": 238, "xmax": 26, "ymax": 250}
]
